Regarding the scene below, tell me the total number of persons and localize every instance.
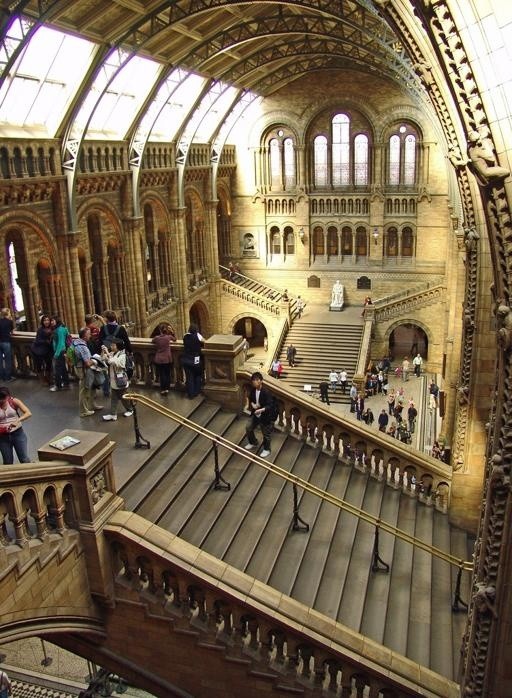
[277,362,283,378]
[319,376,330,405]
[410,332,419,355]
[233,261,244,284]
[0,307,14,381]
[328,367,339,394]
[349,351,441,458]
[363,294,370,305]
[285,343,296,367]
[243,372,274,458]
[242,337,249,362]
[29,308,138,421]
[339,367,348,394]
[330,278,345,306]
[0,386,32,464]
[296,295,304,318]
[263,336,268,351]
[270,358,282,379]
[360,297,373,317]
[228,260,236,283]
[179,321,206,399]
[283,288,289,302]
[150,322,178,395]
[258,361,263,369]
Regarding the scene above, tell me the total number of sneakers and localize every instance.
[92,404,106,411]
[122,410,134,417]
[243,442,259,450]
[48,386,58,392]
[77,409,95,419]
[102,414,118,422]
[259,448,272,458]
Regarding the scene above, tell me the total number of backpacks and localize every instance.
[65,339,87,368]
[102,323,124,353]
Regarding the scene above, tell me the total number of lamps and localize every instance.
[372,226,379,246]
[298,226,305,245]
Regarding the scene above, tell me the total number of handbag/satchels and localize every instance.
[113,371,129,387]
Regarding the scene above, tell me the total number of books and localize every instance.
[49,435,80,451]
